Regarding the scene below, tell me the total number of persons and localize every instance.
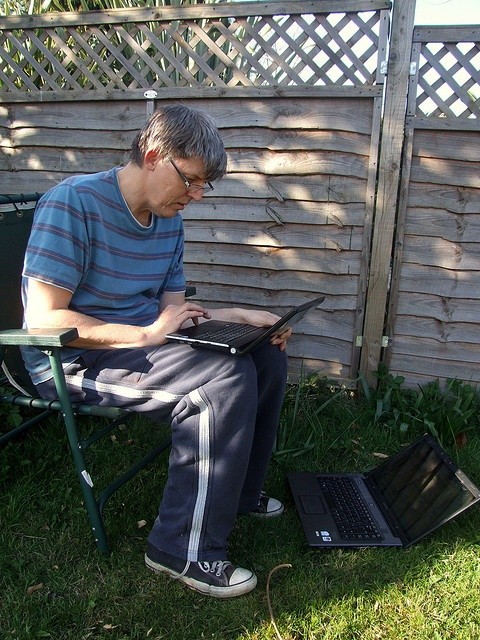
[21,106,293,600]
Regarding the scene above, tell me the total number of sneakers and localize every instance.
[248,495,284,518]
[144,542,258,597]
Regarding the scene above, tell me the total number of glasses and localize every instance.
[169,157,215,197]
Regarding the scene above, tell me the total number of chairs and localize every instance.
[0,193,171,557]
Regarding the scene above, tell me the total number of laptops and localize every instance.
[286,433,479,548]
[161,296,325,353]
